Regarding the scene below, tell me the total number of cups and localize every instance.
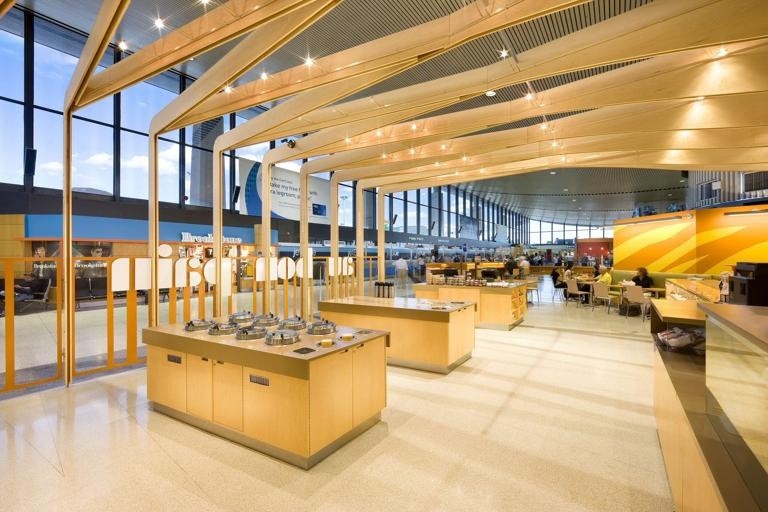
[426,273,487,286]
[744,190,768,199]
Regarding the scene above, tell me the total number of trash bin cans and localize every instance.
[375,282,394,298]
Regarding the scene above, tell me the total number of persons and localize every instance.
[564,264,575,280]
[593,265,600,277]
[520,256,530,267]
[0,270,40,316]
[78,243,107,279]
[582,265,612,305]
[391,256,409,290]
[530,251,613,267]
[631,266,655,286]
[33,244,55,280]
[504,255,519,275]
[416,250,468,276]
[551,267,577,302]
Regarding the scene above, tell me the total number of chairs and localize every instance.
[528,284,539,306]
[375,282,394,297]
[552,277,650,322]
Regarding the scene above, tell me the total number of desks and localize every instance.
[425,262,595,282]
[648,297,707,351]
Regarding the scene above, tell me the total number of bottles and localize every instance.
[374,281,394,298]
